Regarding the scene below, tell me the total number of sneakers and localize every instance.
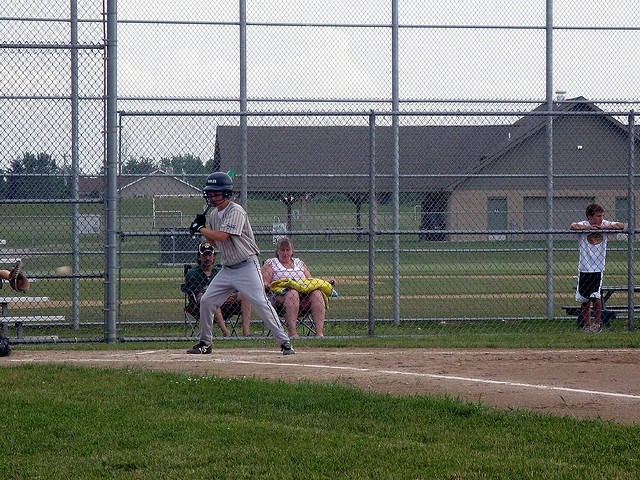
[186,341,212,354]
[582,326,590,331]
[0,341,11,357]
[588,323,602,333]
[281,341,295,354]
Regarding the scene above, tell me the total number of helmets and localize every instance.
[202,172,234,207]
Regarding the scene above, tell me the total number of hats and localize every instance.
[199,242,215,254]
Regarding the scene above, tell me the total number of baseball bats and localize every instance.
[190,169,235,237]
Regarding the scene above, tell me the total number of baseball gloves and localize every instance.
[9,262,29,292]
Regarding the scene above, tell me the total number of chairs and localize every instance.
[262,260,316,336]
[182,262,243,337]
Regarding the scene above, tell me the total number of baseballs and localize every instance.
[58,266,69,277]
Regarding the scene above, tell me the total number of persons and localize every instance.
[260,237,326,338]
[187,243,253,338]
[0,269,22,355]
[570,203,625,334]
[187,172,296,355]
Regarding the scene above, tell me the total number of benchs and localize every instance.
[561,305,640,328]
[571,285,639,308]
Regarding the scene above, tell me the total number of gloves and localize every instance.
[194,214,206,224]
[190,221,204,232]
